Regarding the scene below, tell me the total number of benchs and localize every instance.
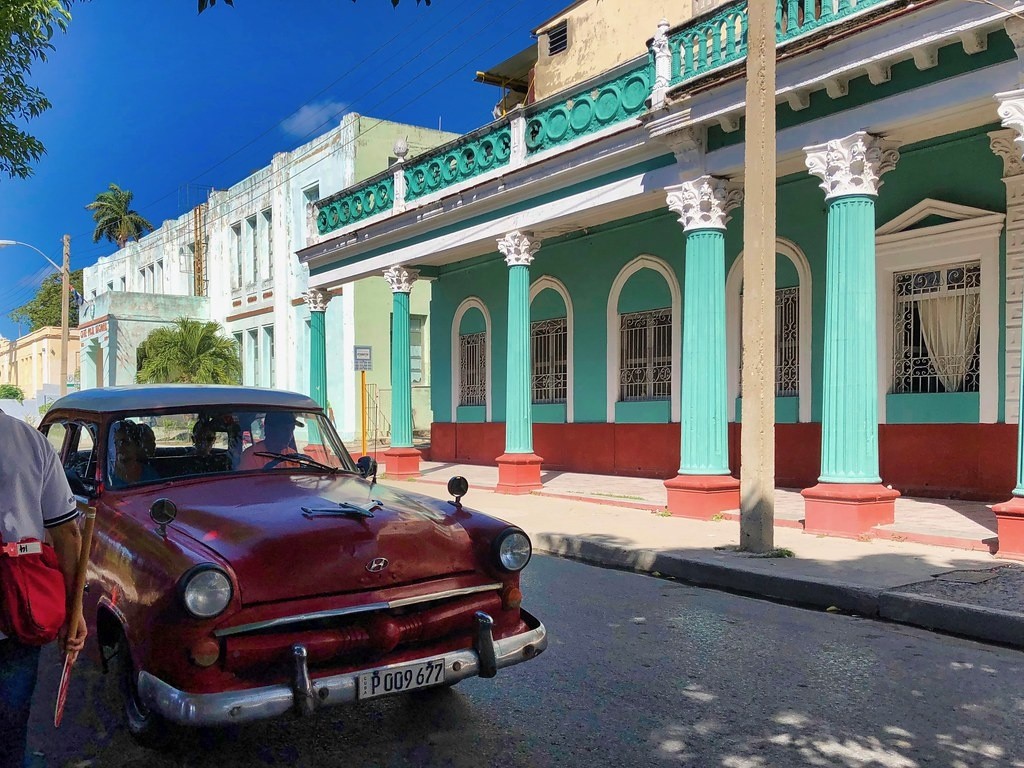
[75,453,232,487]
[69,447,196,469]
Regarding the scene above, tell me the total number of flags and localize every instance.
[73,289,83,305]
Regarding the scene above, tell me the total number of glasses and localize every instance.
[114,438,130,446]
[195,437,214,442]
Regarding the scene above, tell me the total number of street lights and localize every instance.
[0,234,70,398]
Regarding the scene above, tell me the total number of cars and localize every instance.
[37,384,548,748]
[0,399,27,424]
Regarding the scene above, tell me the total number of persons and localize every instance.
[0,409,87,768]
[239,411,305,470]
[173,421,233,477]
[108,420,160,487]
[136,423,156,459]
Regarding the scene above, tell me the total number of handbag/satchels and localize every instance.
[0,537,67,646]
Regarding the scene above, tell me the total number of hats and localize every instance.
[264,411,304,427]
